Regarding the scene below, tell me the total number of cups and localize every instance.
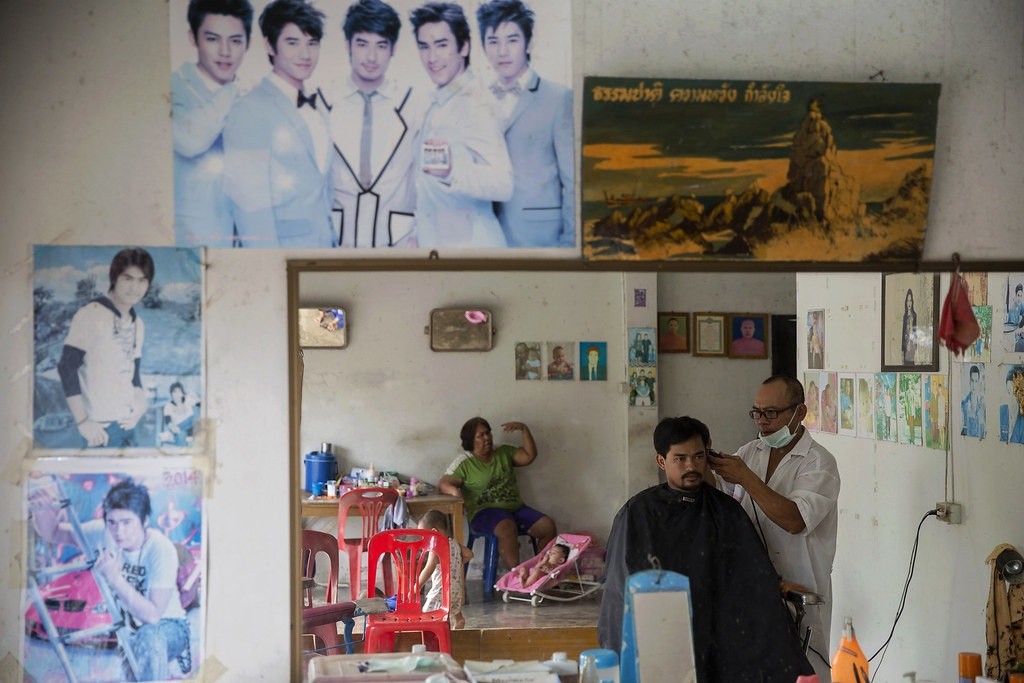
[311,482,325,496]
[321,442,332,454]
[328,480,337,499]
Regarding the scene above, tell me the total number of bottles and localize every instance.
[409,645,426,657]
[828,616,868,683]
[358,465,376,489]
[578,655,601,683]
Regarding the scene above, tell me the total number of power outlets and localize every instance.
[936,502,961,524]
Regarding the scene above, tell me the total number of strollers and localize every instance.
[493,532,602,606]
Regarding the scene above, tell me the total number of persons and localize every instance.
[55,247,196,449]
[28,481,192,682]
[514,343,607,381]
[519,543,570,589]
[407,509,474,629]
[172,0,577,250]
[627,333,657,408]
[440,417,558,570]
[731,318,766,357]
[705,374,841,683]
[597,416,816,683]
[659,318,687,350]
[801,285,1024,448]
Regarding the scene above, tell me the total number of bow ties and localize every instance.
[490,81,522,98]
[297,89,317,109]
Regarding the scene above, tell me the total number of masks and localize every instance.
[759,406,800,449]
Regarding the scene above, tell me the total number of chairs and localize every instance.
[464,519,538,593]
[299,487,453,654]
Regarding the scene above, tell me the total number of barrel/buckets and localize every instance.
[303,451,339,493]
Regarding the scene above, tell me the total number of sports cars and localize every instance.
[24,544,201,650]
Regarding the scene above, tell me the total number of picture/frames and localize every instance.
[658,312,769,360]
[881,272,940,372]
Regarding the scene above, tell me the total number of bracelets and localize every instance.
[76,417,89,425]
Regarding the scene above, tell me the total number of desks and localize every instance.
[301,488,465,606]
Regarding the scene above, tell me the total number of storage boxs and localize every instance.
[308,652,462,683]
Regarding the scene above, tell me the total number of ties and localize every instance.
[357,90,376,190]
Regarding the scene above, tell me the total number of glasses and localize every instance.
[748,403,797,420]
